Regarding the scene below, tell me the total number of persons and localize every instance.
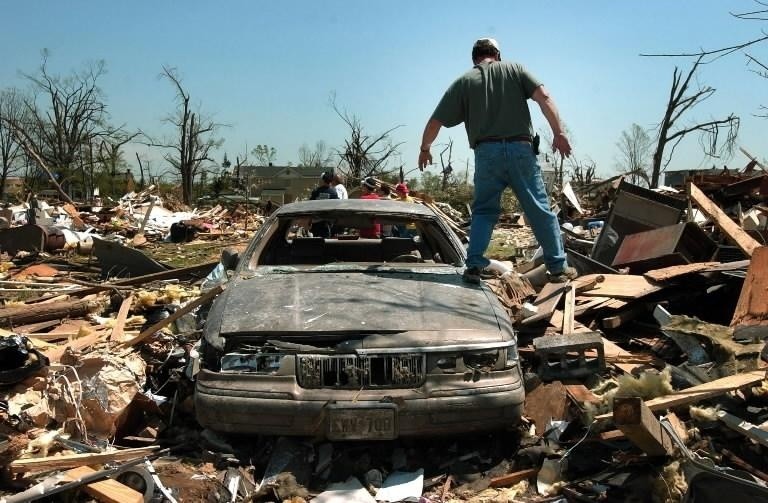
[309,171,348,238]
[418,38,577,285]
[359,177,417,239]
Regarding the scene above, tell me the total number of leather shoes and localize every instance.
[477,135,533,144]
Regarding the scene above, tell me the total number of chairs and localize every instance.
[290,237,337,264]
[379,237,425,264]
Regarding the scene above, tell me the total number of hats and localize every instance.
[473,38,499,51]
[396,184,409,193]
[361,177,376,189]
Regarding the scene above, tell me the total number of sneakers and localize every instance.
[551,267,577,283]
[464,267,480,284]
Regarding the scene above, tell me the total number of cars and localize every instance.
[194,196,527,447]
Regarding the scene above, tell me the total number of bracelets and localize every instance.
[420,146,429,153]
